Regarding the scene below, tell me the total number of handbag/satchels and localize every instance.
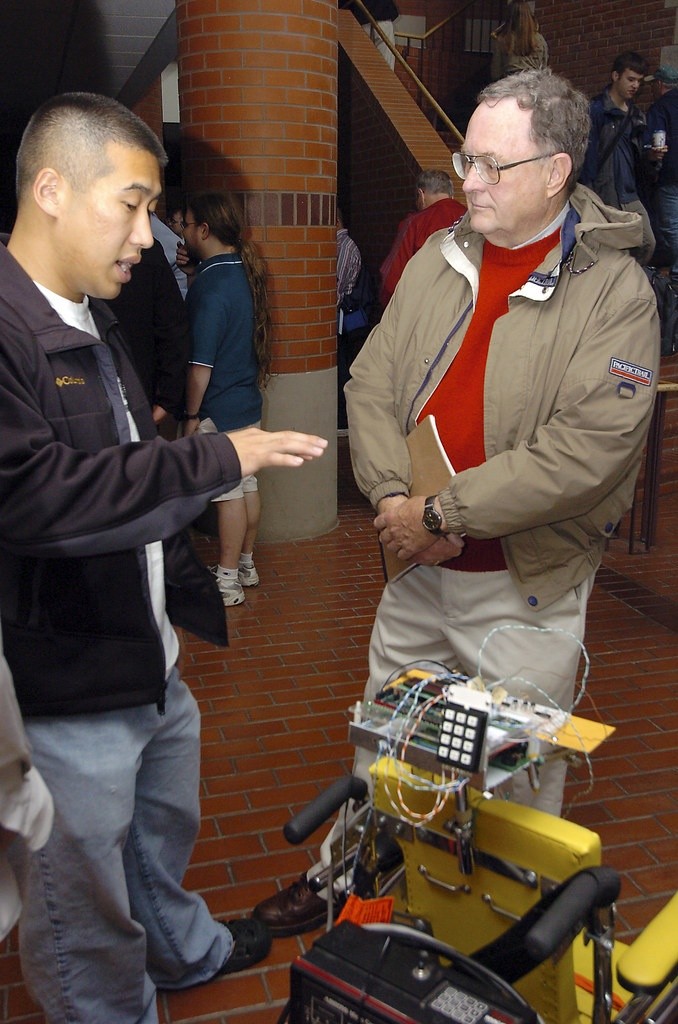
[340,263,376,315]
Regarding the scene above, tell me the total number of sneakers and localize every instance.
[239,561,259,586]
[207,566,245,606]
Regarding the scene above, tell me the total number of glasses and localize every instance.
[452,151,574,185]
[180,222,196,230]
[170,219,178,224]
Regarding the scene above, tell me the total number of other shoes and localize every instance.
[337,429,348,437]
[192,918,273,987]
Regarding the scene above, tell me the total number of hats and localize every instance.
[644,63,678,83]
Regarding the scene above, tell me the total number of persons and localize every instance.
[252,70,661,939]
[1,93,326,1024]
[1,653,55,942]
[105,1,677,606]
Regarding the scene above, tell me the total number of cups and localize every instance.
[653,129,666,147]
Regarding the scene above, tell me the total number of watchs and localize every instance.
[422,494,450,536]
[182,410,199,419]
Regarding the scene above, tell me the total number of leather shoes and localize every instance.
[252,872,342,938]
